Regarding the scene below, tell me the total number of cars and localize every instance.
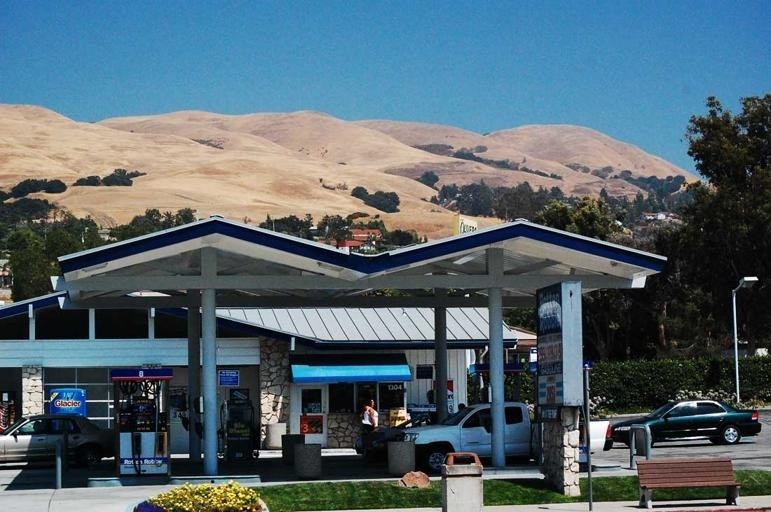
[352,412,437,457]
[0,412,115,469]
[608,399,763,448]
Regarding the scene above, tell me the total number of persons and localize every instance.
[359,399,379,433]
[458,404,465,412]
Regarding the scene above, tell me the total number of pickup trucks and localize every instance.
[404,400,535,474]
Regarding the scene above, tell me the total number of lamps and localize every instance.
[28,304,34,319]
[290,336,296,351]
[150,307,155,317]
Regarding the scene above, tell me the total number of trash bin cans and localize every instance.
[636,424,652,456]
[441,452,484,512]
[281,434,305,465]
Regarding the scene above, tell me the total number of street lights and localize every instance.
[731,275,760,402]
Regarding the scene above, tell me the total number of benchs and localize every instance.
[635,458,742,509]
